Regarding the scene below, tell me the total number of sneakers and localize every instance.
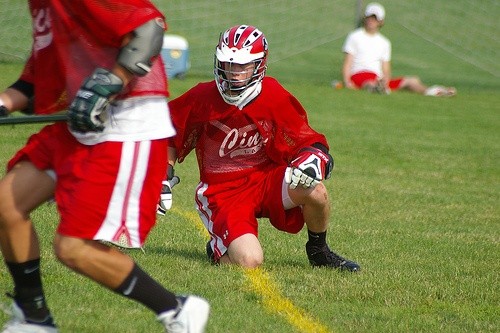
[305,242,360,271]
[0,295,59,333]
[156,296,210,333]
[206,241,220,265]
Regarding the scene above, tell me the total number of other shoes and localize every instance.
[375,79,392,95]
[425,84,455,95]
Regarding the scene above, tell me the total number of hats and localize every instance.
[365,3,387,21]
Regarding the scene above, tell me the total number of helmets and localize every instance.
[214,24,268,91]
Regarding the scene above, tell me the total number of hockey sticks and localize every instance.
[0,110,110,125]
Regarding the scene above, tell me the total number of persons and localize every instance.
[342,1,456,97]
[0,0,214,333]
[156,24,360,272]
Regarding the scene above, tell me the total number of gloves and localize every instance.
[285,146,330,190]
[156,177,181,215]
[67,66,123,133]
